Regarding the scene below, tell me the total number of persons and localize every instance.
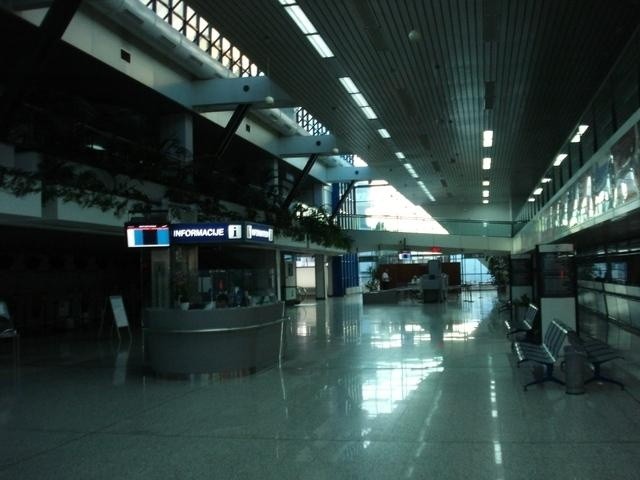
[411,274,420,284]
[203,294,230,309]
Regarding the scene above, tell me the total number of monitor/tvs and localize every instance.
[398,252,411,261]
[124,221,173,250]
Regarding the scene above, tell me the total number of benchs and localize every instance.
[505,303,628,397]
